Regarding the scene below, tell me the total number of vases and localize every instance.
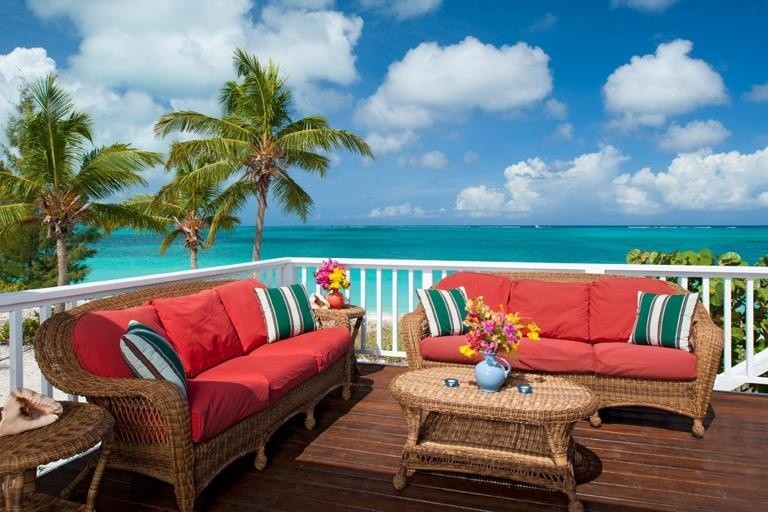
[326,288,344,308]
[473,350,511,393]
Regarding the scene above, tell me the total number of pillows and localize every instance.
[253,283,321,344]
[120,320,187,397]
[628,290,699,353]
[416,285,476,337]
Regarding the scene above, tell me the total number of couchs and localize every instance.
[33,280,351,511]
[398,270,725,437]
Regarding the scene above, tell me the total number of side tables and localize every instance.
[313,304,365,376]
[0,401,115,512]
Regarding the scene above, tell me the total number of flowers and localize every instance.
[312,258,350,292]
[459,297,541,358]
